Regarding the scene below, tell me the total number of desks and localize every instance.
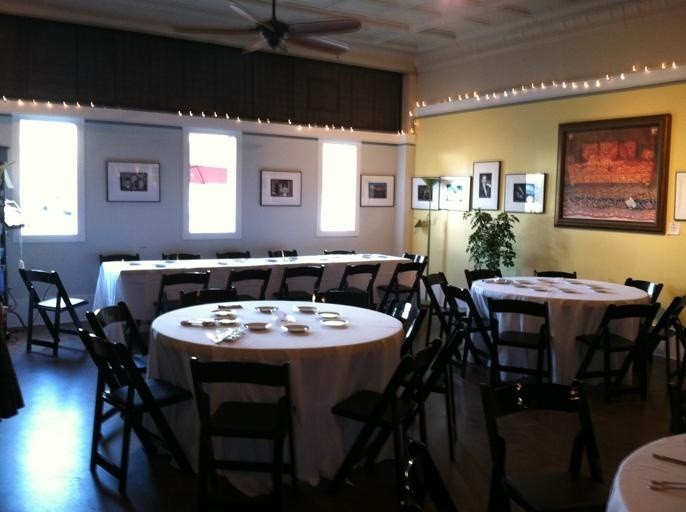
[92,253,416,347]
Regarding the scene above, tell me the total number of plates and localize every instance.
[315,311,342,319]
[539,278,560,286]
[481,277,512,284]
[566,277,587,286]
[587,285,616,295]
[513,282,534,288]
[559,287,584,293]
[243,322,273,330]
[297,306,320,312]
[254,306,278,313]
[515,278,538,285]
[319,319,349,327]
[282,325,310,332]
[532,286,555,293]
[517,279,536,284]
[516,283,528,288]
[210,308,240,316]
[210,316,242,324]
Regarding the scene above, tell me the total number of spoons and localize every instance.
[648,479,686,491]
[588,283,608,294]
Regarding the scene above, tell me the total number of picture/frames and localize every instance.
[259,170,303,207]
[106,159,161,203]
[360,174,395,207]
[554,113,672,235]
[504,172,546,214]
[439,176,473,213]
[411,177,440,211]
[471,161,501,211]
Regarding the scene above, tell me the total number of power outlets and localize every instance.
[666,222,680,235]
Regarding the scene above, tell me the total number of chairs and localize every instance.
[162,251,200,260]
[86,301,147,387]
[376,262,428,322]
[623,278,664,303]
[487,296,553,382]
[399,439,460,512]
[401,327,470,465]
[152,270,211,318]
[220,267,272,302]
[639,294,686,386]
[98,254,141,265]
[401,252,428,309]
[189,356,298,512]
[272,264,325,302]
[18,267,89,357]
[325,249,357,255]
[317,338,443,512]
[439,281,499,382]
[465,268,502,289]
[385,297,428,360]
[215,251,252,259]
[667,315,686,434]
[574,302,661,403]
[267,250,299,257]
[325,262,381,311]
[479,378,611,512]
[78,327,196,495]
[534,270,577,279]
[421,272,466,367]
[180,285,239,307]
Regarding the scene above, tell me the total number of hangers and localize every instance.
[420,177,440,307]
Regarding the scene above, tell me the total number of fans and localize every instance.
[174,1,362,55]
